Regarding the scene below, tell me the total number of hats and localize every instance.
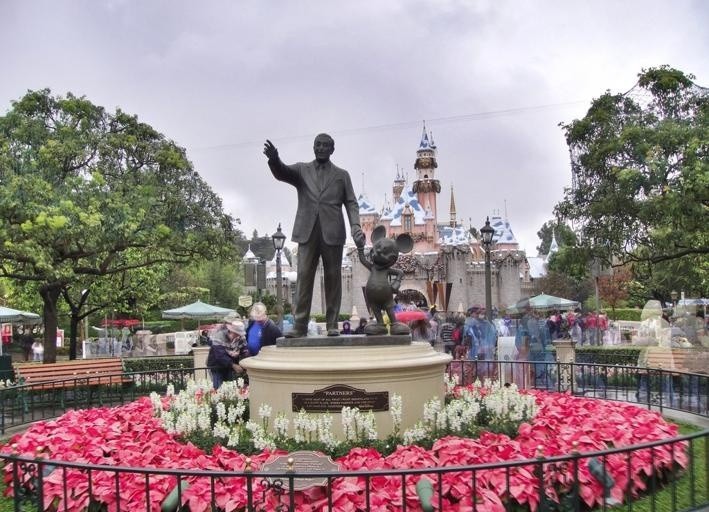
[224,312,246,336]
[250,302,266,317]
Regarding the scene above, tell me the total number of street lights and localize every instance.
[670,290,679,328]
[271,221,287,333]
[479,216,497,322]
[81,287,90,342]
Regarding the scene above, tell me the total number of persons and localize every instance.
[207,319,250,391]
[244,301,283,357]
[355,317,368,334]
[30,337,45,364]
[383,297,708,415]
[262,132,366,340]
[22,330,31,361]
[338,320,355,334]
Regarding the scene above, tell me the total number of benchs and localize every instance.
[13,357,133,414]
[634,348,709,397]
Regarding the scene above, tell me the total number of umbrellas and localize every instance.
[160,301,239,341]
[506,290,582,318]
[0,306,41,357]
